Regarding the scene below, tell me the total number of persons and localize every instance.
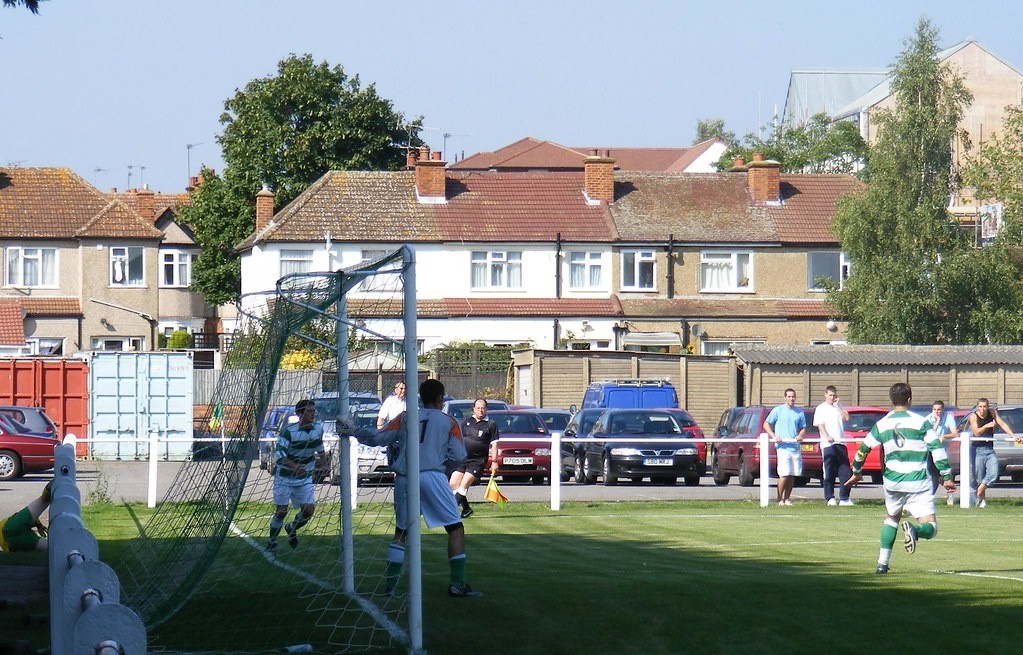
[925,400,957,495]
[968,398,1021,509]
[377,381,420,472]
[449,398,499,518]
[813,386,854,506]
[844,383,957,573]
[336,380,481,597]
[763,389,806,507]
[263,400,331,553]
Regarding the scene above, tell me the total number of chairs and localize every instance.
[613,421,627,432]
[643,422,671,434]
[862,418,873,428]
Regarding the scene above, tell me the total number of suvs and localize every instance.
[943,402,1022,482]
[712,404,823,487]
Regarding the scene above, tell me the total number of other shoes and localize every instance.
[776,498,792,505]
[827,498,837,507]
[975,496,986,508]
[839,497,854,506]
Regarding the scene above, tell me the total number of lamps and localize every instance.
[101,318,108,328]
[357,318,365,327]
[582,320,590,328]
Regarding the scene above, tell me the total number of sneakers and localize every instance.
[901,521,918,554]
[461,507,473,518]
[448,583,483,597]
[384,588,402,597]
[285,523,298,548]
[264,543,279,552]
[875,562,890,574]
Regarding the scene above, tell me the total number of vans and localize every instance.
[571,380,679,438]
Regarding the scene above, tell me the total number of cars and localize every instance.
[0,405,60,478]
[442,396,571,436]
[472,411,552,486]
[582,410,700,487]
[329,409,393,484]
[652,407,707,474]
[712,407,747,454]
[259,392,384,473]
[841,406,892,484]
[561,408,607,481]
[909,404,971,426]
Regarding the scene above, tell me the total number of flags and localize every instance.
[209,401,224,431]
[485,479,508,512]
